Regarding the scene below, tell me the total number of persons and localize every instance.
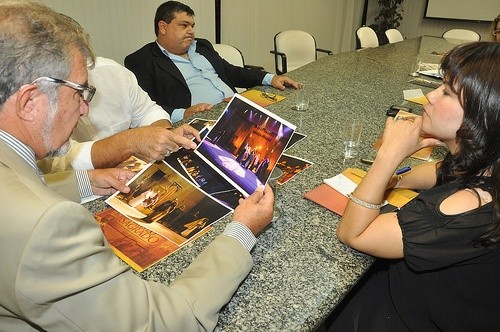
[124,0,301,123]
[36,57,202,173]
[323,41,500,332]
[0,0,273,332]
[491,15,500,42]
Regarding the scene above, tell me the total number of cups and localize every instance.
[409,55,423,76]
[339,123,363,159]
[293,81,310,111]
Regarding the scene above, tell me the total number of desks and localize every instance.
[83,36,473,332]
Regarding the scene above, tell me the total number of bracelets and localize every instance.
[348,193,382,209]
[394,174,402,188]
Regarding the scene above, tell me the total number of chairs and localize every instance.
[212,43,266,96]
[269,28,333,78]
[355,25,480,50]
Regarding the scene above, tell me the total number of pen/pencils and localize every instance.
[397,166,411,175]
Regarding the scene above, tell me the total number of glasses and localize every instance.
[31,76,96,102]
[493,30,500,35]
